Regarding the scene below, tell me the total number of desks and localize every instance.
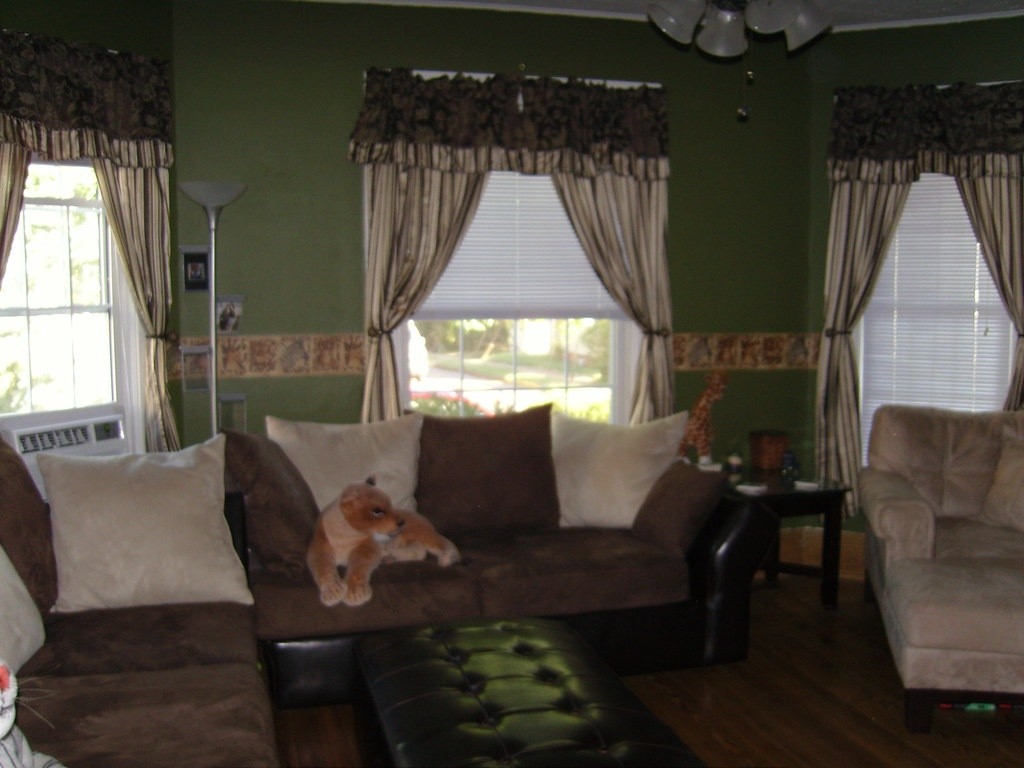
[739,475,854,613]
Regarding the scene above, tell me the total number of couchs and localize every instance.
[17,498,783,768]
[857,408,1024,734]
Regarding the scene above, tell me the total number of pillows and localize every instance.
[218,427,321,588]
[547,410,688,531]
[33,431,255,614]
[629,459,731,559]
[262,410,425,513]
[402,404,561,538]
[0,545,47,677]
[0,433,58,612]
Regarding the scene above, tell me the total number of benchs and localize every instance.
[350,616,707,768]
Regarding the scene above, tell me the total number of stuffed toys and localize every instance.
[308,475,462,607]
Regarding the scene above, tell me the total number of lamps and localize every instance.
[179,181,249,438]
[647,0,835,61]
